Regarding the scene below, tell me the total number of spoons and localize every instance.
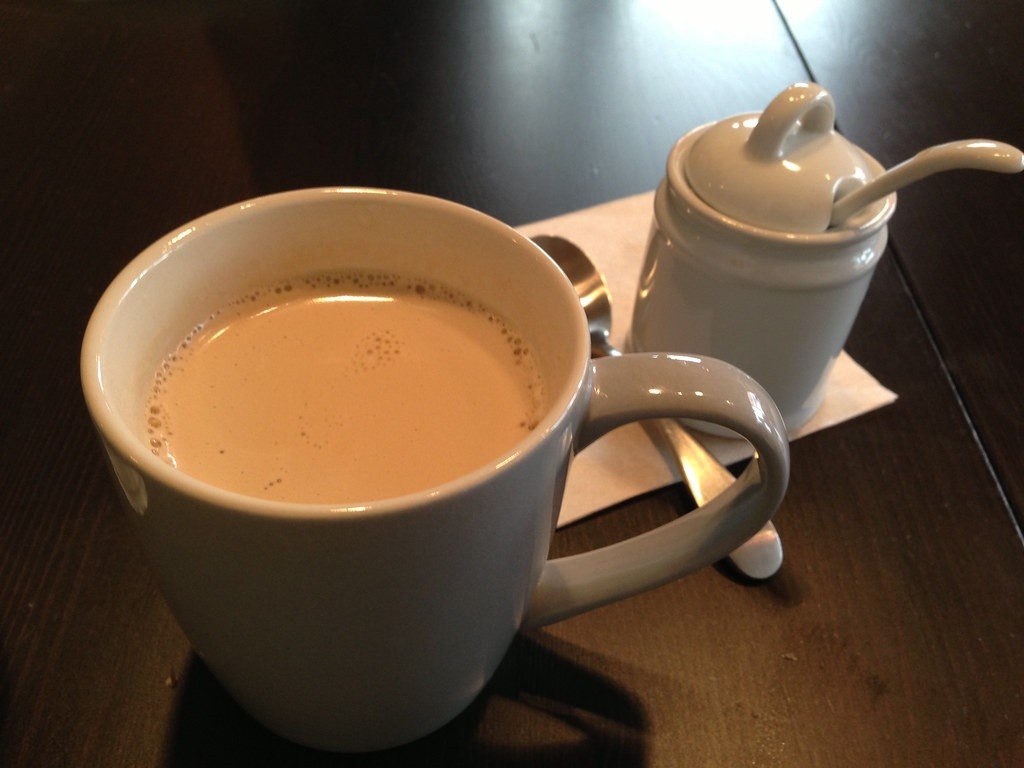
[527,235,783,580]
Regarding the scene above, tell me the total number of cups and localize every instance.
[80,187,791,748]
[632,80,897,438]
